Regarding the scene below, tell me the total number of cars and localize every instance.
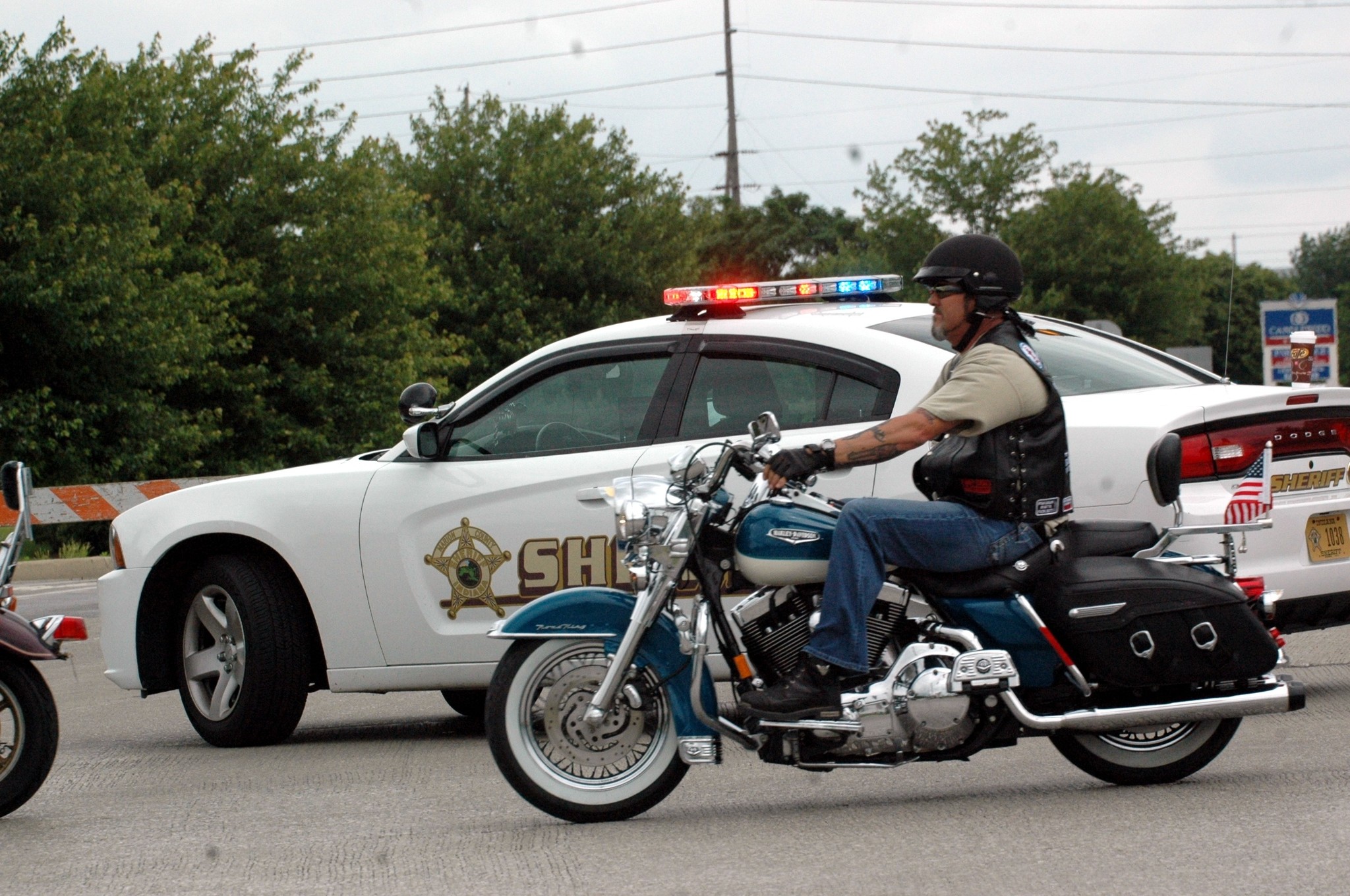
[92,268,1349,740]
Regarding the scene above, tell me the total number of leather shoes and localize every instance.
[740,657,844,722]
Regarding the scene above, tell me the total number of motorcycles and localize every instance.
[466,400,1305,828]
[0,459,84,823]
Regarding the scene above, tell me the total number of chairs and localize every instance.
[696,366,758,434]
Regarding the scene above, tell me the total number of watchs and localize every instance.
[820,438,837,472]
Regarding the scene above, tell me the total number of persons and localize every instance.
[737,234,1073,720]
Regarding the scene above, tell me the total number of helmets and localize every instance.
[912,234,1024,309]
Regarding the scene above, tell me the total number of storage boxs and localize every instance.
[1042,556,1279,691]
